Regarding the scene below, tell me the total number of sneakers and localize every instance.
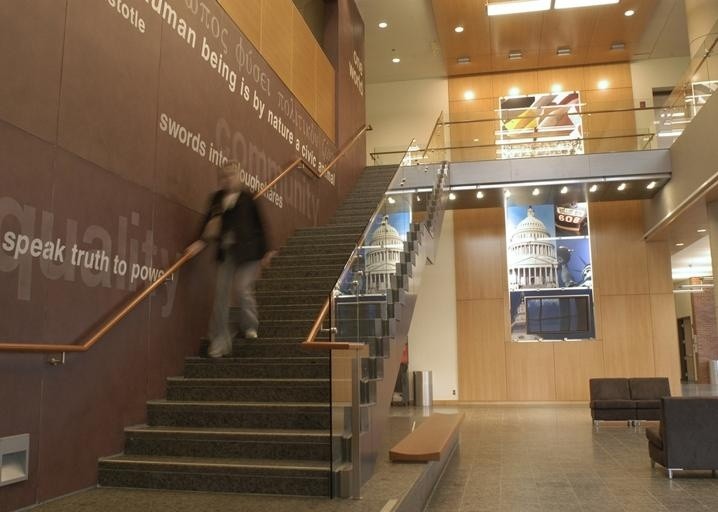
[247,327,258,340]
[209,350,223,358]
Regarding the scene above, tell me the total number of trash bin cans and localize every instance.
[414,371,433,406]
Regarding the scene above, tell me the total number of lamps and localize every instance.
[484,0,621,17]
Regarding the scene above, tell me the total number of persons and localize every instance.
[185,162,276,358]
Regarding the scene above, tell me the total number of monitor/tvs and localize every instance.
[524,294,592,334]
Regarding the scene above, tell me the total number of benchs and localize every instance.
[389,411,464,462]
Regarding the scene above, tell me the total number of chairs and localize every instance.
[590,378,635,426]
[646,398,718,479]
[629,377,670,424]
